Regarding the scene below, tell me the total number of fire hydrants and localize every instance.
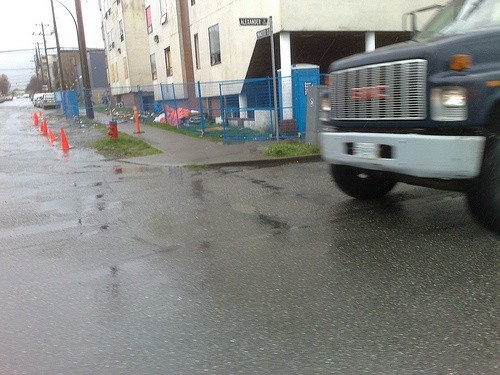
[107,118,120,140]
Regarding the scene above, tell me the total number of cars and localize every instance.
[31,92,57,111]
[0,93,12,104]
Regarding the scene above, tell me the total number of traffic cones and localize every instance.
[33,111,50,136]
[47,127,59,143]
[59,127,76,151]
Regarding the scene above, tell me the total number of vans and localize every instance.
[316,0,500,238]
